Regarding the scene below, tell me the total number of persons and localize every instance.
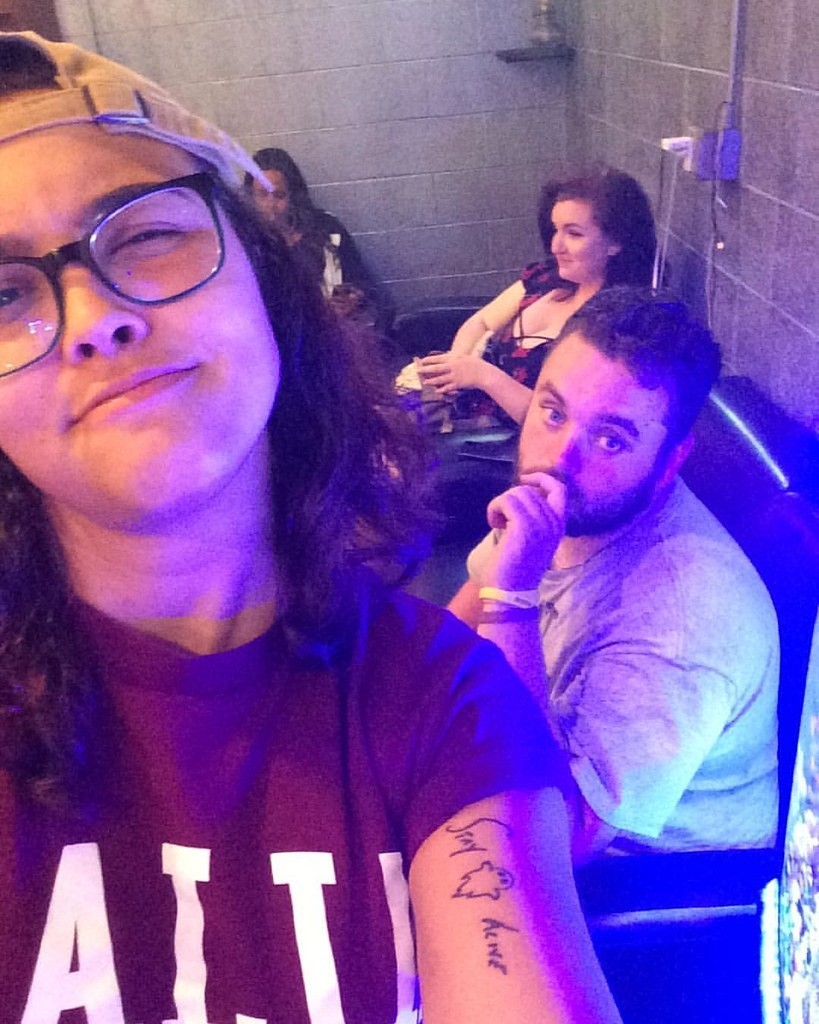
[395,160,657,435]
[241,147,395,350]
[441,287,780,872]
[0,29,620,1023]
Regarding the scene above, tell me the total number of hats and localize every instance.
[0,29,276,197]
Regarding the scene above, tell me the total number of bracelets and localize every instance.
[476,587,541,624]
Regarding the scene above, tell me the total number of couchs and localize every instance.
[383,293,819,1024]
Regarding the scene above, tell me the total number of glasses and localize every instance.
[0,173,223,379]
